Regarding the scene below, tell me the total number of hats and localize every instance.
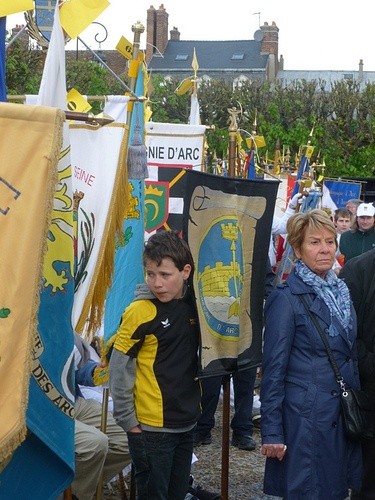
[357,203,375,216]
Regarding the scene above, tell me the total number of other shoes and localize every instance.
[191,476,221,500]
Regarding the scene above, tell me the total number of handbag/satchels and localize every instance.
[340,384,375,441]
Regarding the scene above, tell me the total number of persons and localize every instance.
[63,199,375,500]
[260,209,362,500]
[108,230,202,500]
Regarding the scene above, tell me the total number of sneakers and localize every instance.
[199,428,213,444]
[231,434,256,449]
